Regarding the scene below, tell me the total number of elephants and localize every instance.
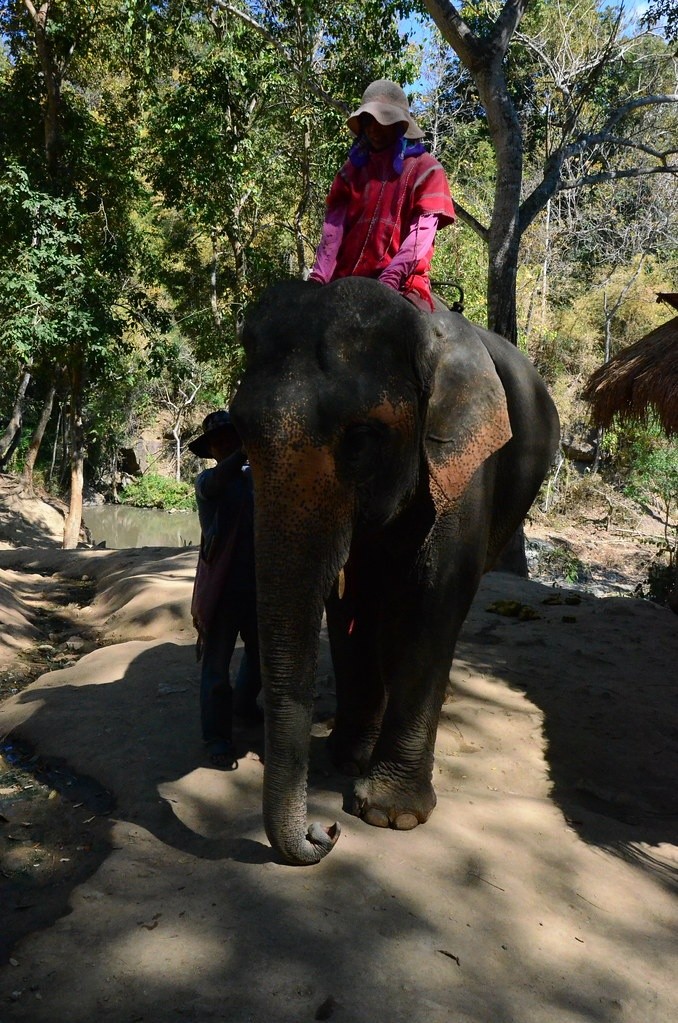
[229,276,559,867]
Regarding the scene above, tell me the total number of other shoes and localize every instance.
[205,741,236,768]
[234,701,265,722]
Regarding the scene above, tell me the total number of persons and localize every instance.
[308,78,454,313]
[188,411,264,766]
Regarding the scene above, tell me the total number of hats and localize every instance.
[188,411,236,459]
[346,80,427,140]
